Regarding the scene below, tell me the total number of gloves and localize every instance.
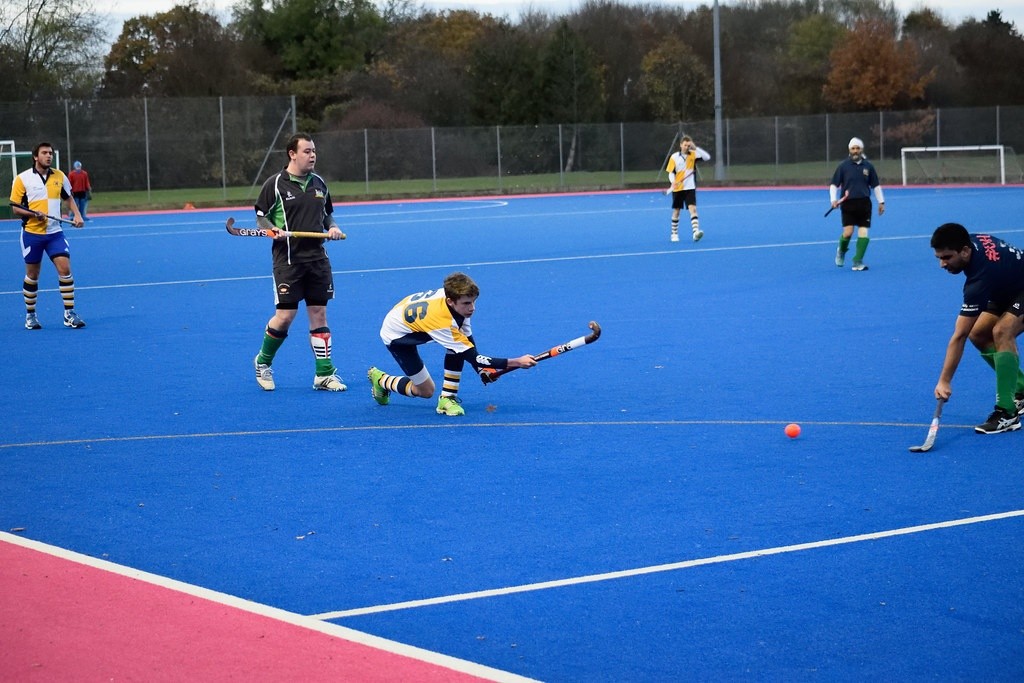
[477,366,500,384]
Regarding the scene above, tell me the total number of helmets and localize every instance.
[74,161,82,168]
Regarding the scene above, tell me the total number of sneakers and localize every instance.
[836,246,846,266]
[313,368,348,392]
[975,405,1022,434]
[851,262,868,270]
[63,309,86,328]
[693,230,704,242]
[436,394,465,416]
[670,233,680,242]
[1012,393,1024,414]
[26,312,43,330]
[254,353,275,391]
[367,367,392,406]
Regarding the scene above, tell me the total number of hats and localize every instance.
[848,136,864,153]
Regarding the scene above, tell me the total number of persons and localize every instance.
[255,133,348,392]
[931,223,1024,434]
[828,137,884,271]
[10,143,85,330]
[666,136,711,242]
[368,271,539,417]
[68,161,92,221]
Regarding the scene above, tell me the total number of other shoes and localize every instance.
[82,217,90,221]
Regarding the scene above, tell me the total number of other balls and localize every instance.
[784,424,801,438]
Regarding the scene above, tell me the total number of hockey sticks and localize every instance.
[225,216,347,240]
[907,396,944,452]
[824,189,849,218]
[662,170,695,196]
[8,202,77,225]
[481,320,601,384]
